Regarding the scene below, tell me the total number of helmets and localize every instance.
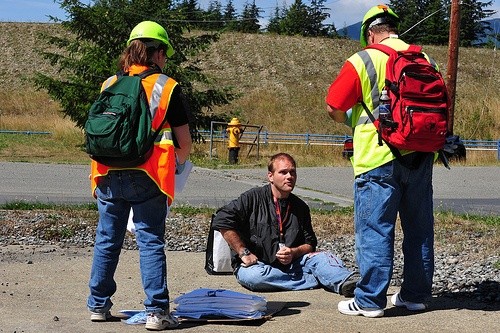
[360,4,399,48]
[127,21,174,59]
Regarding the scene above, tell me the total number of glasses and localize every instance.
[164,53,169,63]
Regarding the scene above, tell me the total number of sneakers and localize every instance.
[342,274,362,297]
[338,298,384,318]
[90,310,112,321]
[145,311,179,331]
[391,292,428,310]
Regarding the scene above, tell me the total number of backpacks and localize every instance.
[361,43,449,151]
[84,69,168,168]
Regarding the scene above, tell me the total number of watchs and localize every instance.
[239,248,250,258]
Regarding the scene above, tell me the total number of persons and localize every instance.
[327,5,440,318]
[225,118,244,163]
[87,20,192,330]
[211,153,359,297]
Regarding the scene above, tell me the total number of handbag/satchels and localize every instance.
[204,214,234,275]
